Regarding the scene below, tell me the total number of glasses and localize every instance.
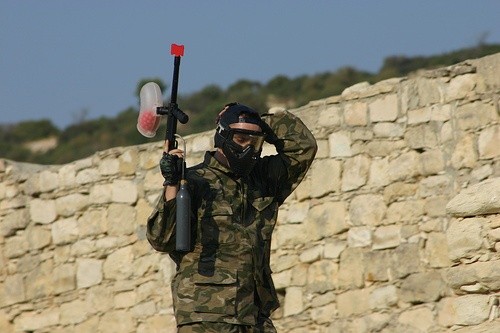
[219,122,264,153]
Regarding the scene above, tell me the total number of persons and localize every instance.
[146,102,319,333]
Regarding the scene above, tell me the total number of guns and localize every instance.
[137,43,192,251]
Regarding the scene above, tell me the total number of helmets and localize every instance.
[213,102,266,147]
